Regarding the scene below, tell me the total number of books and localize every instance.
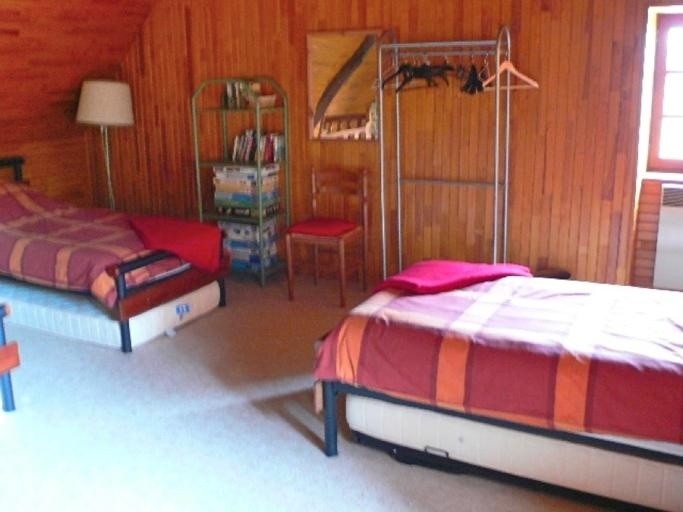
[208,81,282,160]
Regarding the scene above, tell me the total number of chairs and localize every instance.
[284,167,369,310]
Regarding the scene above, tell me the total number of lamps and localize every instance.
[76,80,136,212]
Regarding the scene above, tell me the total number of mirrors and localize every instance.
[305,28,383,142]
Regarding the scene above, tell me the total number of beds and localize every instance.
[315,269,683,512]
[0,156,228,352]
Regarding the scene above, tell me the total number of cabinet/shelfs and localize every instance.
[191,76,293,290]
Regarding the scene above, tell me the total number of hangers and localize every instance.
[371,49,540,95]
